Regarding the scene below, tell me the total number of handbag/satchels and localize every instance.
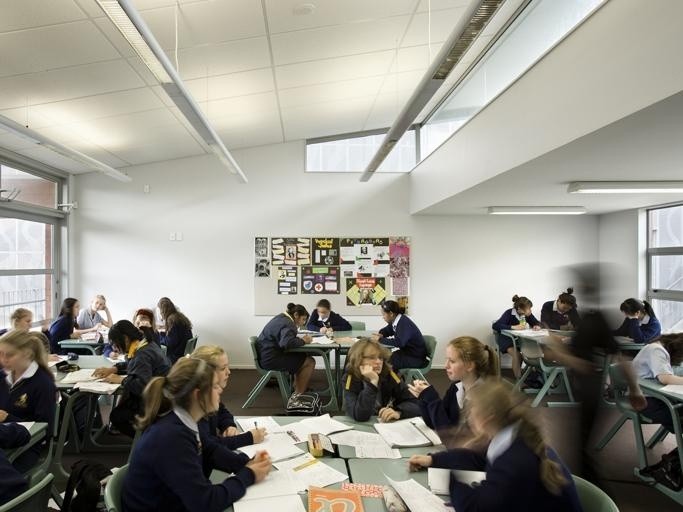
[521,369,545,389]
[640,456,682,492]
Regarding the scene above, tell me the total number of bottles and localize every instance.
[67,352,79,371]
[519,313,526,326]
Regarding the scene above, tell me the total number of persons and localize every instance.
[252,300,316,394]
[627,331,683,494]
[359,246,367,254]
[408,336,502,448]
[46,297,83,355]
[537,286,579,330]
[104,297,197,436]
[490,291,550,388]
[367,299,429,373]
[124,342,274,510]
[0,330,59,480]
[285,246,295,260]
[76,295,116,328]
[403,374,578,511]
[1,307,34,340]
[604,299,663,395]
[541,259,648,505]
[339,337,422,423]
[0,420,42,511]
[304,298,352,333]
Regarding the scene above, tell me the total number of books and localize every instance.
[228,414,490,511]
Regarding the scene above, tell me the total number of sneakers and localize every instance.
[602,388,618,405]
[287,392,311,409]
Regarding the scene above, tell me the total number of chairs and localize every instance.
[0,335,198,510]
[491,320,681,511]
[242,320,436,410]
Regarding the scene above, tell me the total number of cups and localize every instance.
[307,433,324,457]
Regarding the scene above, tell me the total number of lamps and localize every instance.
[566,181,682,193]
[487,206,587,215]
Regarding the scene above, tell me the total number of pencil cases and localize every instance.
[308,433,335,457]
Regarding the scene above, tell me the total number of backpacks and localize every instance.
[61,464,113,512]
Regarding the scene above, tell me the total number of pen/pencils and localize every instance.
[254,422,258,430]
[323,323,326,328]
